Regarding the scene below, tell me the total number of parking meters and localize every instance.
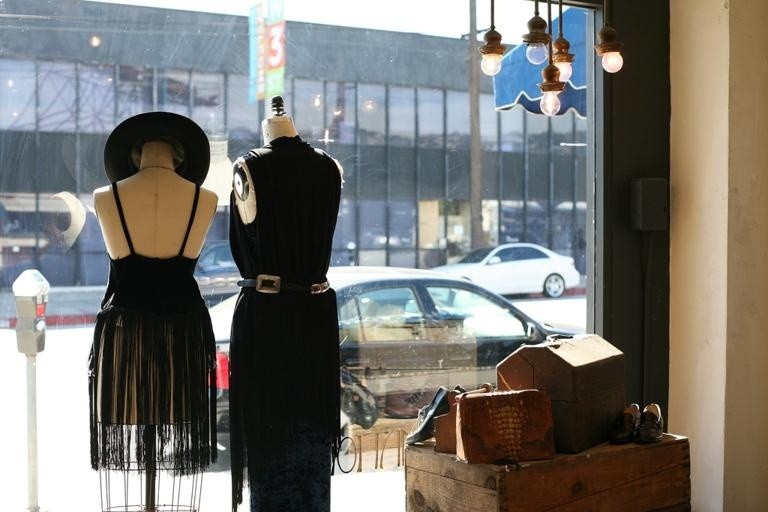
[12,268,53,512]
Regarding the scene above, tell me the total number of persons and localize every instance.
[223,108,347,512]
[85,111,221,476]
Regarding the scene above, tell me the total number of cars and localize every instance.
[207,265,575,450]
[360,223,404,250]
[194,237,244,302]
[427,243,580,298]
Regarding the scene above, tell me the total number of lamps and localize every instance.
[477,1,626,119]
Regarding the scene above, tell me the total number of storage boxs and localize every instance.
[495,332,629,455]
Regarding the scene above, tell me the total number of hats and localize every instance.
[104,111,210,187]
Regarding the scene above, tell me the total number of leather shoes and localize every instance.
[405,386,468,445]
[384,389,436,417]
[352,398,378,429]
[637,402,664,443]
[609,403,640,444]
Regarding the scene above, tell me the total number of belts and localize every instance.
[237,276,329,297]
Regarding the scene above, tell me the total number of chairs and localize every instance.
[338,299,405,345]
